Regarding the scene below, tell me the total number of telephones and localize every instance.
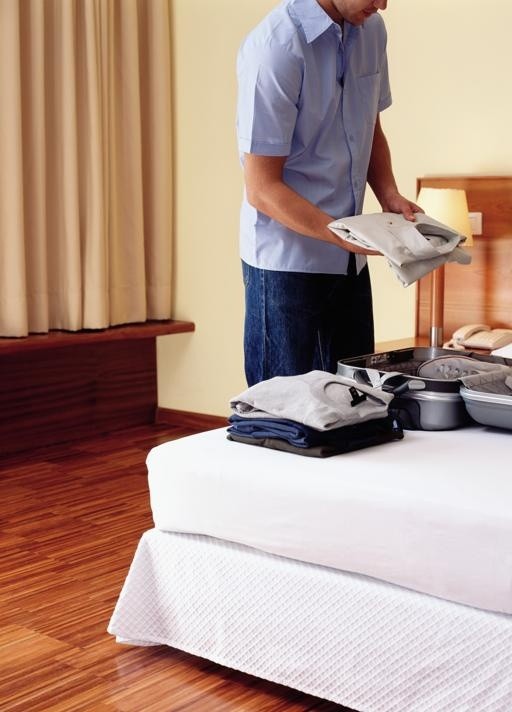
[452,324,512,350]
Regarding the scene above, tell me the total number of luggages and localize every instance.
[336,328,512,431]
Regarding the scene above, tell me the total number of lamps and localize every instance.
[412,188,474,361]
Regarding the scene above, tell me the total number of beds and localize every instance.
[105,176,512,712]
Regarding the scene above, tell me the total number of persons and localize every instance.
[235,0,425,387]
